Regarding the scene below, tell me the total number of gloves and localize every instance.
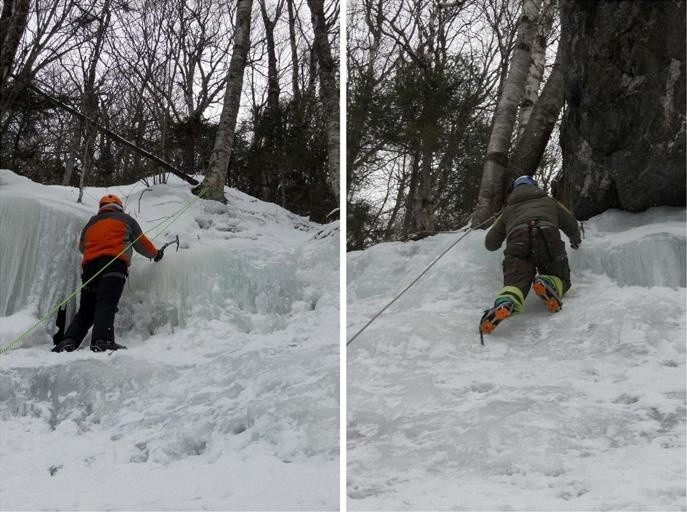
[154,251,165,261]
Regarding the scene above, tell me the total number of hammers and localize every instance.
[161,234,180,252]
[570,222,585,248]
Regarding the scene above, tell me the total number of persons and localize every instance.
[50,194,163,353]
[478,175,581,334]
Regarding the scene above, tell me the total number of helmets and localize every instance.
[513,175,537,186]
[100,195,122,208]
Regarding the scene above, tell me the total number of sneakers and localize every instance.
[91,340,127,352]
[51,339,75,352]
[532,277,562,312]
[480,301,513,333]
